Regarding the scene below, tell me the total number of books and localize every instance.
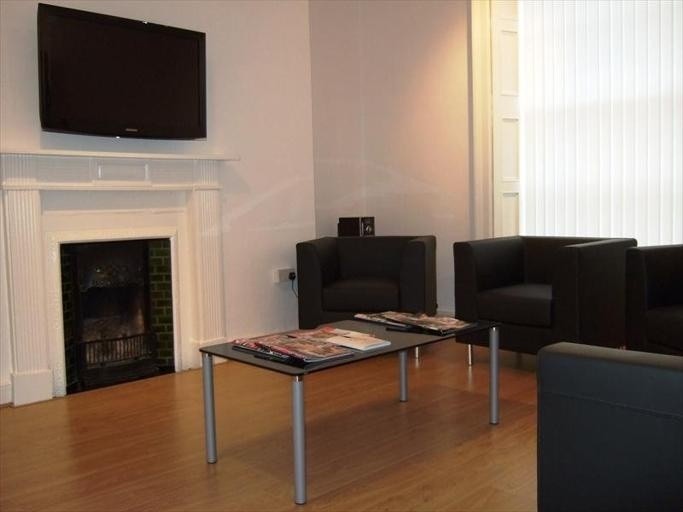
[230,310,477,365]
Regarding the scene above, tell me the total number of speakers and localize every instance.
[338,216,375,237]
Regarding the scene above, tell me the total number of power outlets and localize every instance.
[274,267,296,283]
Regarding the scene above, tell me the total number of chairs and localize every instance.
[296,236,436,328]
[536,341,682,512]
[453,234,637,366]
[626,243,682,355]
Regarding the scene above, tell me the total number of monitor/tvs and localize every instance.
[36,3,206,141]
